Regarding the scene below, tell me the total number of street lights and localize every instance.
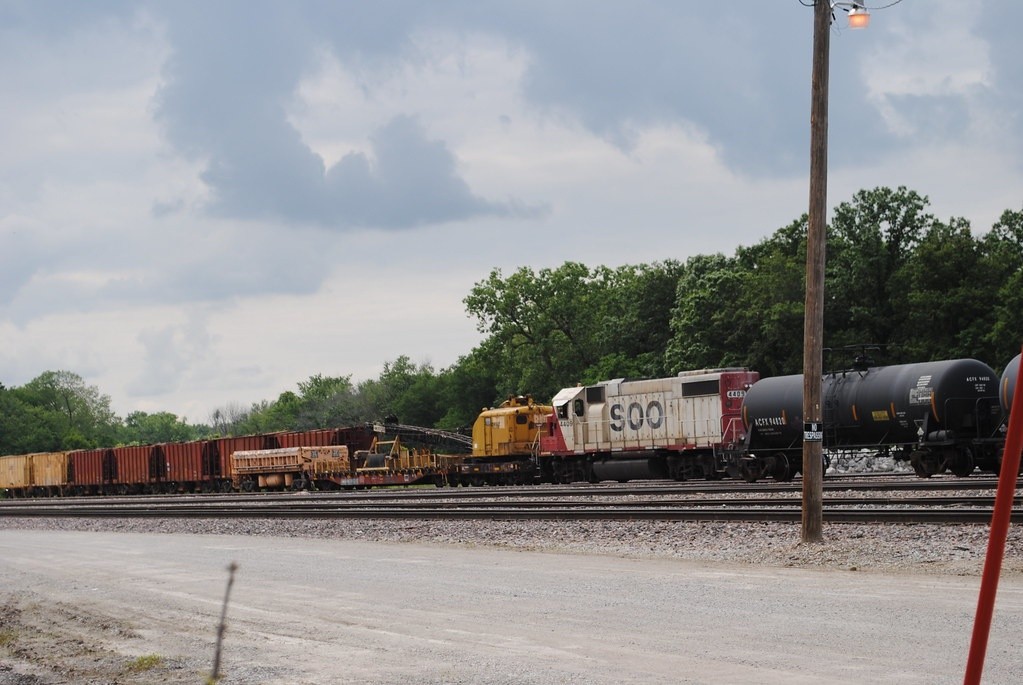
[800,0,873,544]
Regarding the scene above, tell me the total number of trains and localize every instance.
[4,395,554,487]
[536,344,1023,485]
